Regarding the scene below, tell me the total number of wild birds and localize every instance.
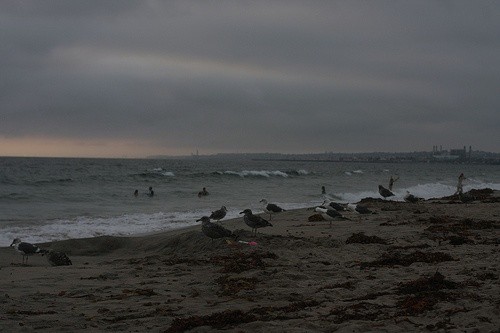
[320,199,357,214]
[209,205,228,221]
[9,236,51,265]
[403,189,424,203]
[238,209,274,236]
[198,186,209,199]
[379,180,395,200]
[135,186,154,196]
[259,198,288,222]
[196,215,232,243]
[314,206,355,227]
